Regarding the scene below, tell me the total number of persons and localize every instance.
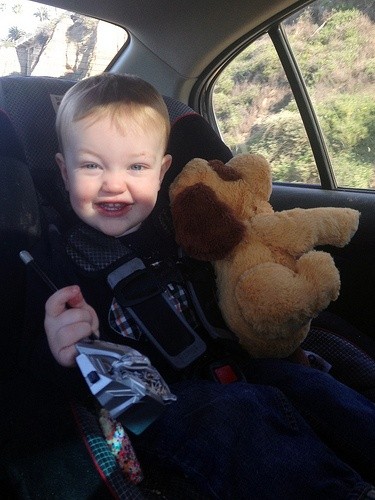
[0,71,374,500]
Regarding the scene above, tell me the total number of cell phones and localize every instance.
[129,293,208,370]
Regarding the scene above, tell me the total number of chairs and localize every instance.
[2,74,374,500]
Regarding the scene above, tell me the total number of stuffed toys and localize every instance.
[167,152,362,360]
